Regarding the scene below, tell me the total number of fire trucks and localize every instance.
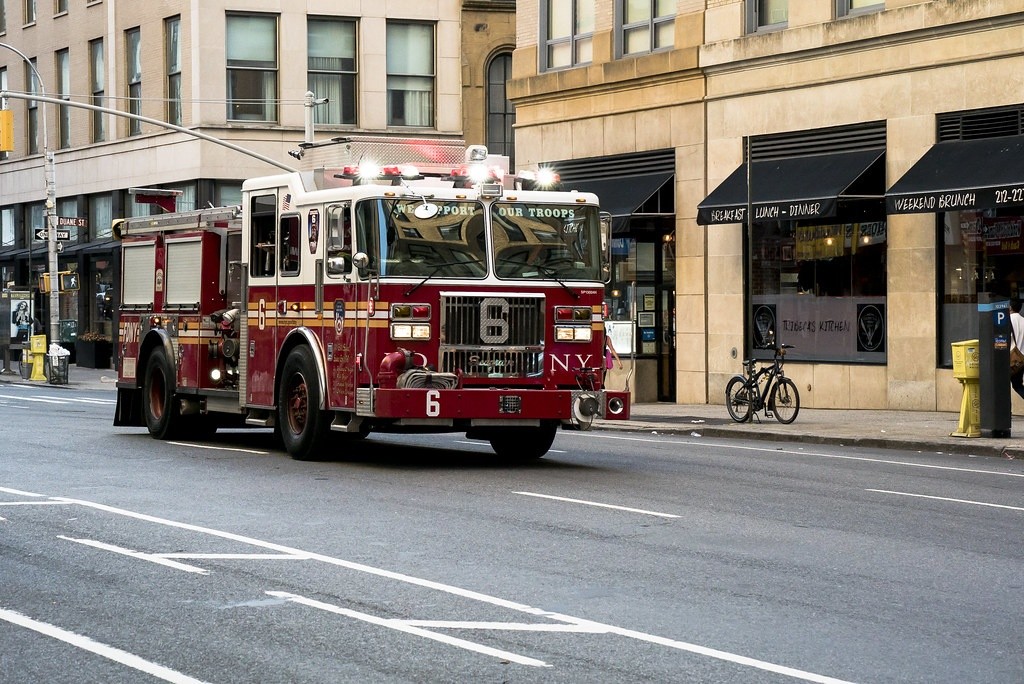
[107,128,636,462]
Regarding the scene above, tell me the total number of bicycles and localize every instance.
[725,331,801,424]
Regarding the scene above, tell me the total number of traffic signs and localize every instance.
[57,215,88,227]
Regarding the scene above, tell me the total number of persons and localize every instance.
[309,224,318,254]
[1007,296,1024,399]
[600,327,624,389]
[12,301,34,344]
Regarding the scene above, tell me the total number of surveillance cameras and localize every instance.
[314,98,329,105]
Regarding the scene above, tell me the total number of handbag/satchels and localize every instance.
[605,349,613,369]
[1010,346,1024,375]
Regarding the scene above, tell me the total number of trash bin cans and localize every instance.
[46,353,69,386]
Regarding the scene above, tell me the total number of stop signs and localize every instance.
[35,228,72,241]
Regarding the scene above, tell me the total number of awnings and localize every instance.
[0,239,122,260]
[696,149,886,225]
[882,134,1024,215]
[514,173,673,234]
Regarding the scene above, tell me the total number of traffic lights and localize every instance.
[60,272,81,292]
[36,276,51,295]
